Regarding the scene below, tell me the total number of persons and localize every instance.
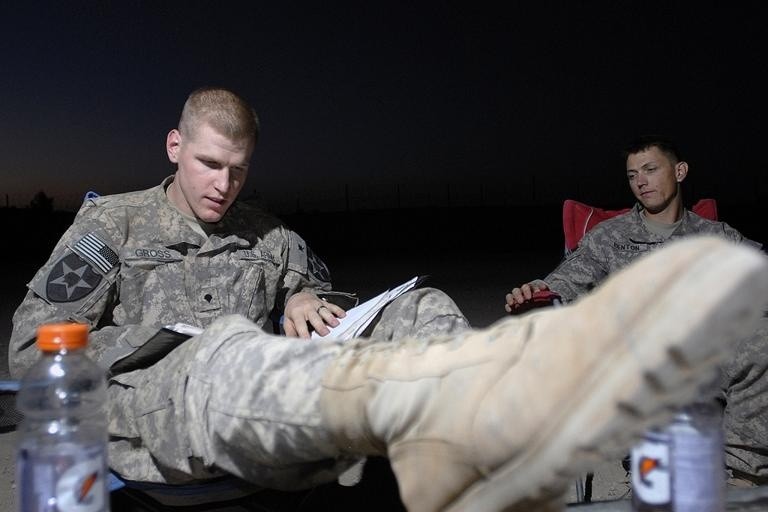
[503,135,768,510]
[7,86,765,511]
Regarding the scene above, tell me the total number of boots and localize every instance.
[323,234,767,511]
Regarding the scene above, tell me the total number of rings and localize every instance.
[316,306,328,314]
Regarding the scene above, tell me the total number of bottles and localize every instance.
[14,321,112,512]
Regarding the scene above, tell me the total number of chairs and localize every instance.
[508,198,716,316]
